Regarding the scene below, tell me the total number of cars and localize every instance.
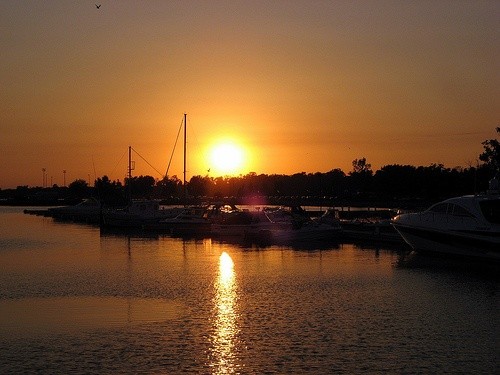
[388,194,499,233]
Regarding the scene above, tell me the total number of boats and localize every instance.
[23,198,342,240]
